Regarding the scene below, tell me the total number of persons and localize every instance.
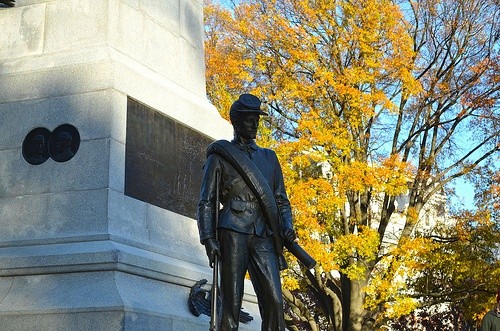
[483,290,500,331]
[194,93,296,331]
